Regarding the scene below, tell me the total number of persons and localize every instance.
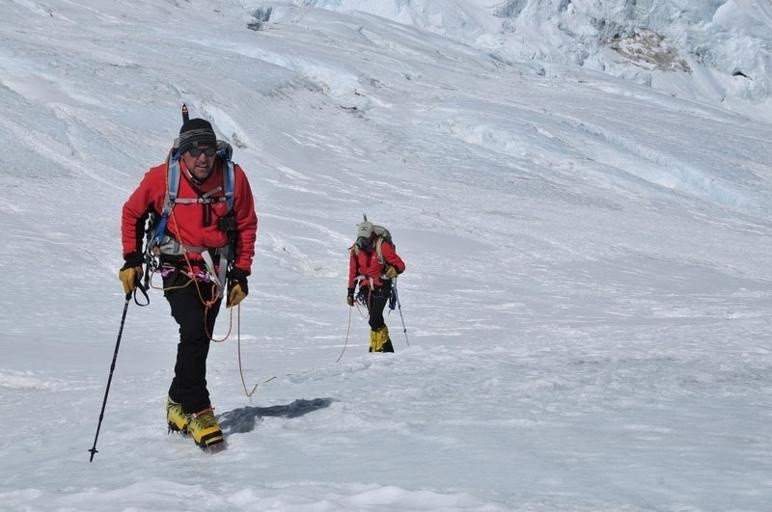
[119,118,258,449]
[346,221,405,354]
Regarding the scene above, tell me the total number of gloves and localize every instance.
[385,265,398,278]
[119,258,146,294]
[226,266,249,309]
[347,293,356,306]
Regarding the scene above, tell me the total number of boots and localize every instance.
[369,326,393,353]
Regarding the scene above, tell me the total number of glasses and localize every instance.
[187,146,217,157]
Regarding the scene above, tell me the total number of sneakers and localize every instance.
[165,393,191,431]
[187,407,224,450]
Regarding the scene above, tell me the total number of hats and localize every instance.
[178,118,217,154]
[358,221,375,238]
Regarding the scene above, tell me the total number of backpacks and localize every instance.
[354,225,395,268]
[155,137,236,220]
